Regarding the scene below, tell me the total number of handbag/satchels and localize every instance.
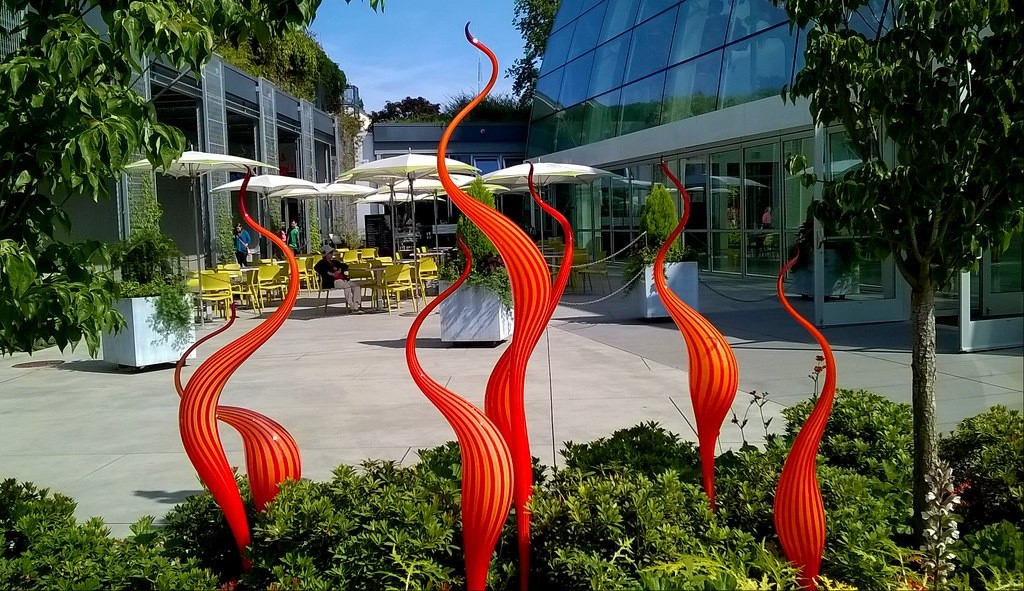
[247,248,250,255]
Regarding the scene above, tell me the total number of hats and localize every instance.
[320,245,334,257]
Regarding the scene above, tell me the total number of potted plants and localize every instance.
[617,181,698,322]
[100,176,198,373]
[439,177,513,346]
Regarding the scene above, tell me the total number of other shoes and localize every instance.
[352,309,364,314]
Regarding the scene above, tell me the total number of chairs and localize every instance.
[186,246,441,321]
[536,237,613,298]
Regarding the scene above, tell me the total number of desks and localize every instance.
[433,247,452,265]
[344,266,415,312]
[395,259,419,264]
[544,255,564,286]
[409,253,445,258]
[240,266,285,309]
[206,275,239,320]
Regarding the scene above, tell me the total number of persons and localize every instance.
[286,219,301,255]
[271,225,287,261]
[233,223,251,267]
[314,244,366,315]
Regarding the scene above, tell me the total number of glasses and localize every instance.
[329,251,333,254]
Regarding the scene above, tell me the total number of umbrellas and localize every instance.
[123,143,281,328]
[324,146,545,259]
[456,156,620,261]
[209,169,319,257]
[566,172,770,251]
[260,178,380,252]
[783,157,879,181]
